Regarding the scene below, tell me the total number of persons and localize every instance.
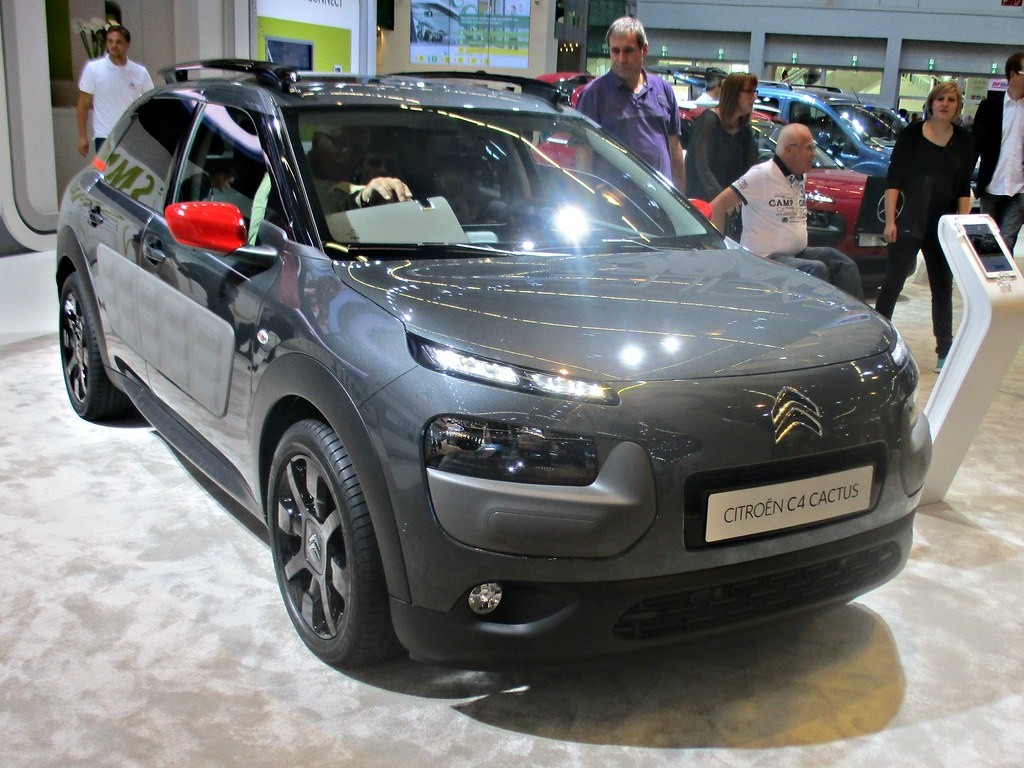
[873,81,971,374]
[960,115,974,131]
[77,24,155,159]
[567,16,686,203]
[178,166,238,202]
[694,67,728,103]
[684,72,760,238]
[429,152,527,242]
[708,122,865,306]
[898,109,920,125]
[298,117,414,214]
[970,52,1024,259]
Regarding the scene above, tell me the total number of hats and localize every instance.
[704,67,729,79]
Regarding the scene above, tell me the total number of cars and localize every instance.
[534,64,941,261]
[54,52,939,674]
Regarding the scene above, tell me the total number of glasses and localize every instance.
[743,89,758,94]
[315,131,364,156]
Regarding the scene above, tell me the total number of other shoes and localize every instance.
[935,354,948,373]
